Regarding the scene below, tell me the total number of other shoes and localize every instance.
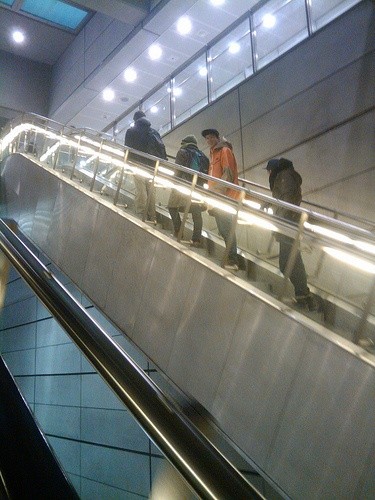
[223,256,239,271]
[190,239,202,249]
[170,232,184,239]
[293,295,319,312]
[145,214,157,224]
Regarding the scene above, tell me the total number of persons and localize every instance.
[201,129,241,269]
[124,110,160,226]
[263,156,319,312]
[167,135,210,247]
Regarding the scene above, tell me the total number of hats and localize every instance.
[133,110,146,120]
[181,134,200,146]
[262,158,279,169]
[201,129,220,138]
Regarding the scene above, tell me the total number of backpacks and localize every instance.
[134,125,168,161]
[185,147,209,175]
